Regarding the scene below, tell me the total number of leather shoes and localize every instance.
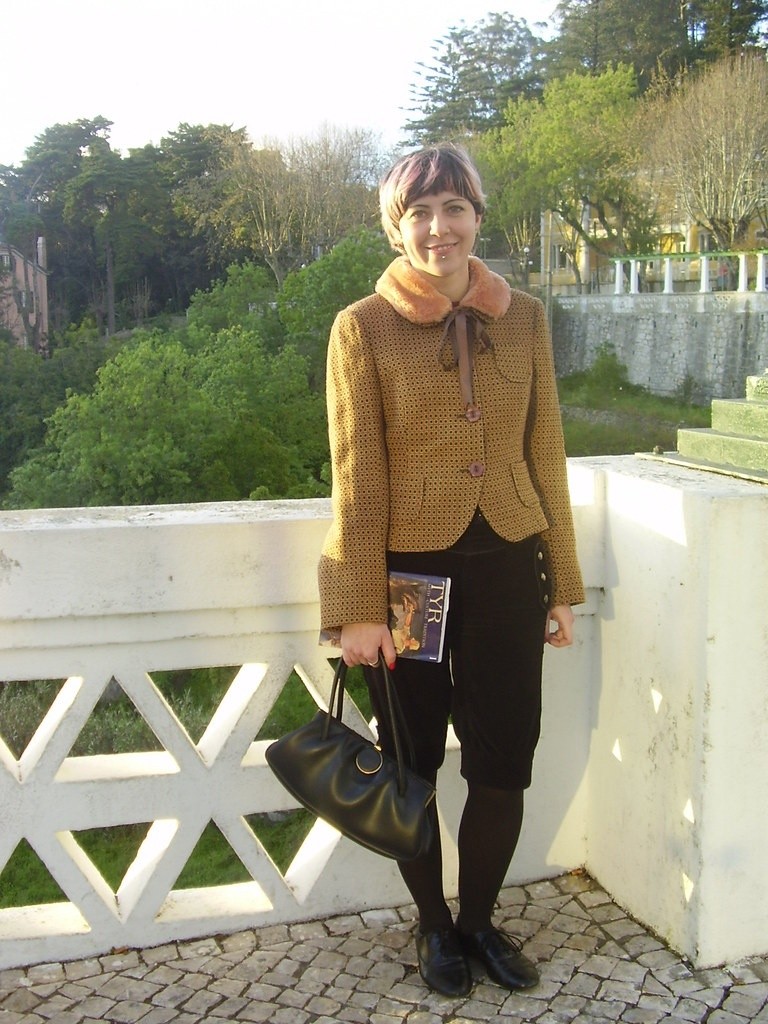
[454,913,540,990]
[415,920,473,997]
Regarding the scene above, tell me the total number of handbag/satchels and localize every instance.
[265,652,437,863]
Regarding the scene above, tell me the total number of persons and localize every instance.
[321,142,585,997]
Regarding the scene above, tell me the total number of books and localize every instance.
[317,570,451,664]
